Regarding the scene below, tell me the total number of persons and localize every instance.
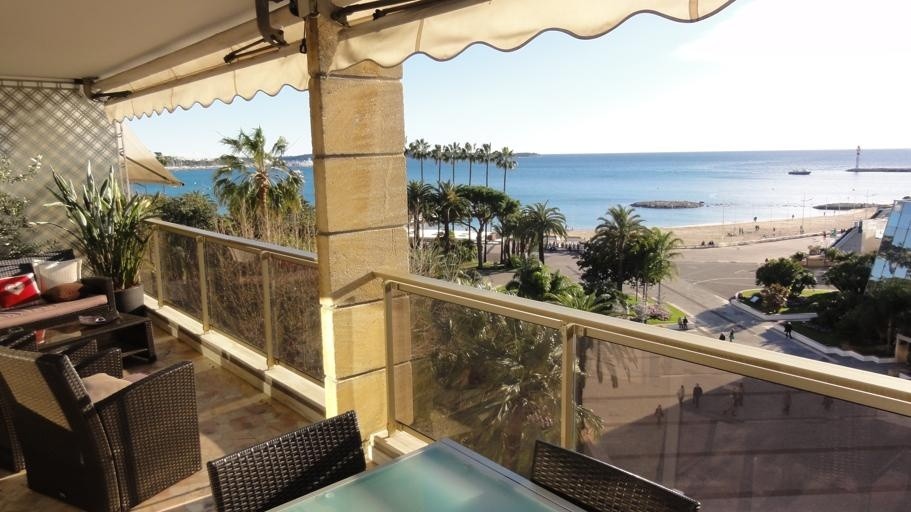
[678,316,684,328]
[653,404,664,425]
[677,384,686,408]
[732,387,739,408]
[784,321,793,339]
[782,391,793,415]
[720,332,726,341]
[722,391,736,417]
[729,327,737,343]
[739,382,745,406]
[693,382,703,409]
[684,316,689,329]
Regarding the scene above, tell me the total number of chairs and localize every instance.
[529,440,701,512]
[0,328,98,473]
[207,409,367,512]
[0,348,202,512]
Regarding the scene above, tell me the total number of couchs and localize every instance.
[0,249,116,337]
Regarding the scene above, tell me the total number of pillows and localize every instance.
[0,258,83,311]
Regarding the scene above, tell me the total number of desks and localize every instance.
[263,437,586,512]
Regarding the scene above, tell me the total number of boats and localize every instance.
[788,168,811,175]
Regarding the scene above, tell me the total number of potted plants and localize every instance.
[31,159,158,316]
